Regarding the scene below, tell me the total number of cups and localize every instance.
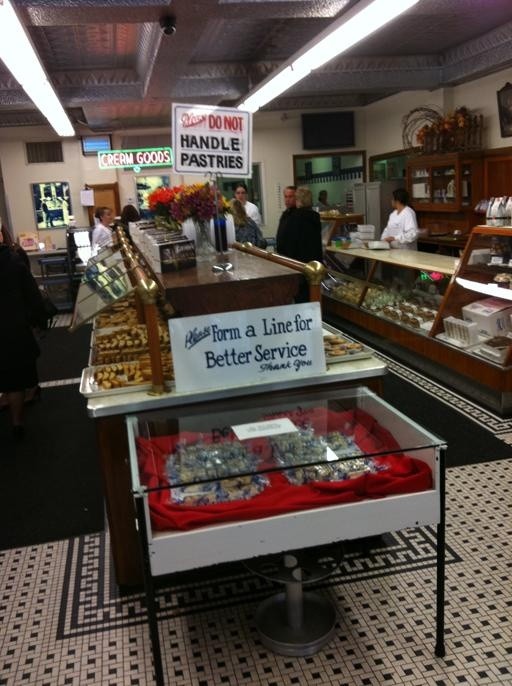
[328,209,336,217]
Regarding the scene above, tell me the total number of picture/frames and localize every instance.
[29,180,74,229]
[496,80,512,137]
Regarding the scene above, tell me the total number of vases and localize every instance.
[191,218,222,264]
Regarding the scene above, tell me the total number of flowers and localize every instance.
[147,179,223,225]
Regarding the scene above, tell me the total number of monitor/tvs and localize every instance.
[300,111,356,150]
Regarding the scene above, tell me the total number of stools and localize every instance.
[38,255,71,300]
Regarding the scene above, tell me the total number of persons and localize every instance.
[92,207,113,255]
[318,191,330,209]
[231,181,262,230]
[380,188,419,251]
[279,189,322,303]
[0,223,42,391]
[276,185,297,256]
[229,199,263,248]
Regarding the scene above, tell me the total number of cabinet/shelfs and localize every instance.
[67,225,390,591]
[128,390,446,684]
[404,153,478,212]
[322,224,512,421]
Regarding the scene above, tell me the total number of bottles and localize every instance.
[447,179,455,198]
